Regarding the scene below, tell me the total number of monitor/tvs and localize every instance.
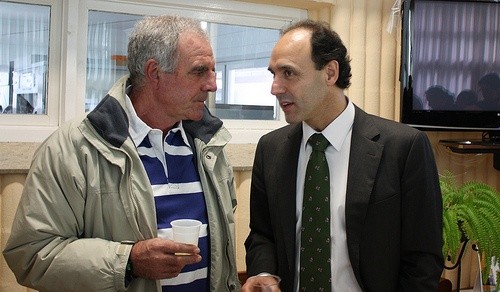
[400,0,500,134]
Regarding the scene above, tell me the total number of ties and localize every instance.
[300,133,333,292]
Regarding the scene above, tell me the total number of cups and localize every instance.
[170,218,202,264]
[247,275,281,292]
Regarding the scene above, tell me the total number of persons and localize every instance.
[424,72,500,111]
[241,18,447,292]
[2,14,242,292]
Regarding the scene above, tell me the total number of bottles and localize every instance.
[482,272,496,292]
